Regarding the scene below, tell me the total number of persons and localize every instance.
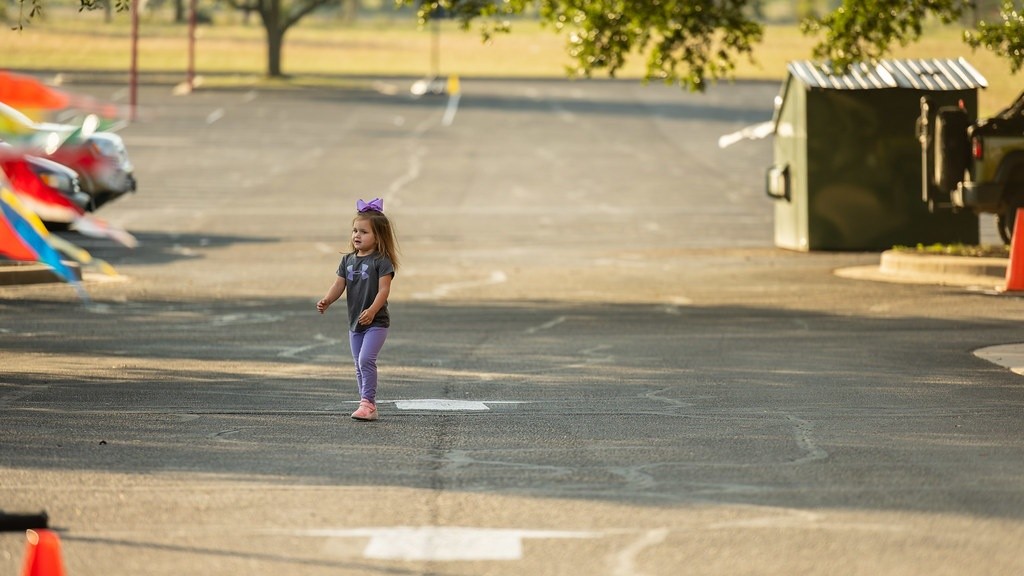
[317,199,401,420]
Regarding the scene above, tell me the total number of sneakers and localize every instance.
[351,399,378,421]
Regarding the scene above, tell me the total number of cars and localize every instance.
[1,99,140,206]
[0,138,95,230]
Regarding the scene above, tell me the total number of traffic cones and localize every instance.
[1000,204,1023,292]
[11,527,64,576]
